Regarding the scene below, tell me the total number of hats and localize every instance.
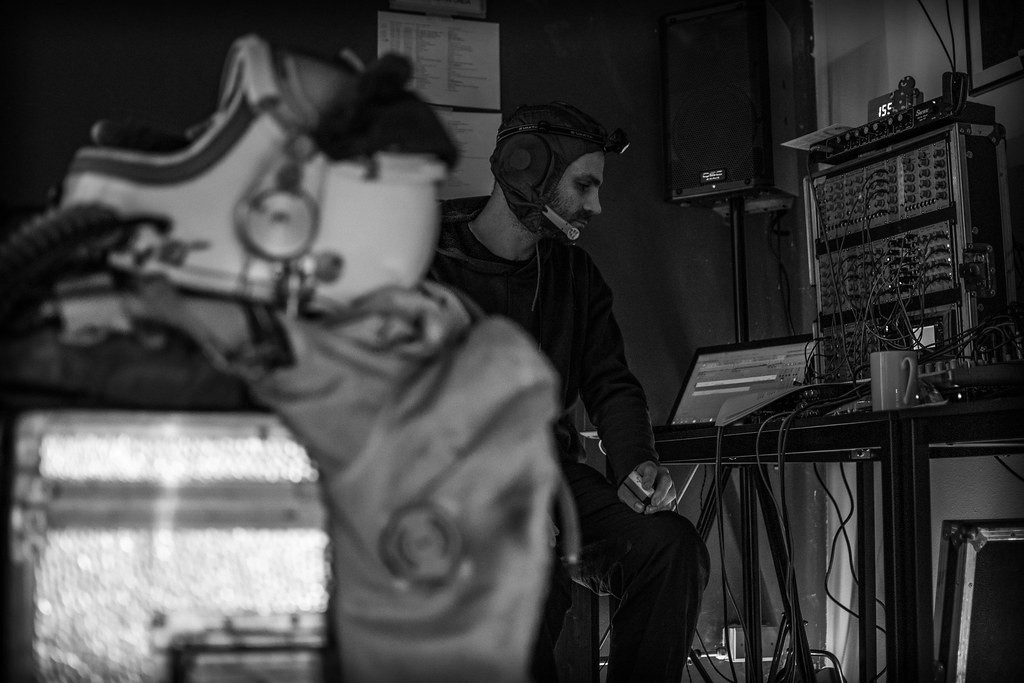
[492,101,611,244]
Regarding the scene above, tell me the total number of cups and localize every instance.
[869,349,919,411]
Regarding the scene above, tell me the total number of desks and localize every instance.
[598,406,1024,683]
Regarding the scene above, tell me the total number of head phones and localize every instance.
[497,102,608,204]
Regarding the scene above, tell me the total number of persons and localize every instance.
[422,102,710,683]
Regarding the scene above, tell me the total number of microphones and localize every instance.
[541,203,580,241]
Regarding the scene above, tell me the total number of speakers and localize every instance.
[659,1,801,217]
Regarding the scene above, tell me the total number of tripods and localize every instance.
[694,191,820,683]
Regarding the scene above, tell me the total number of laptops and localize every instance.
[577,333,817,441]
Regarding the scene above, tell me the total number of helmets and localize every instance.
[54,46,452,308]
